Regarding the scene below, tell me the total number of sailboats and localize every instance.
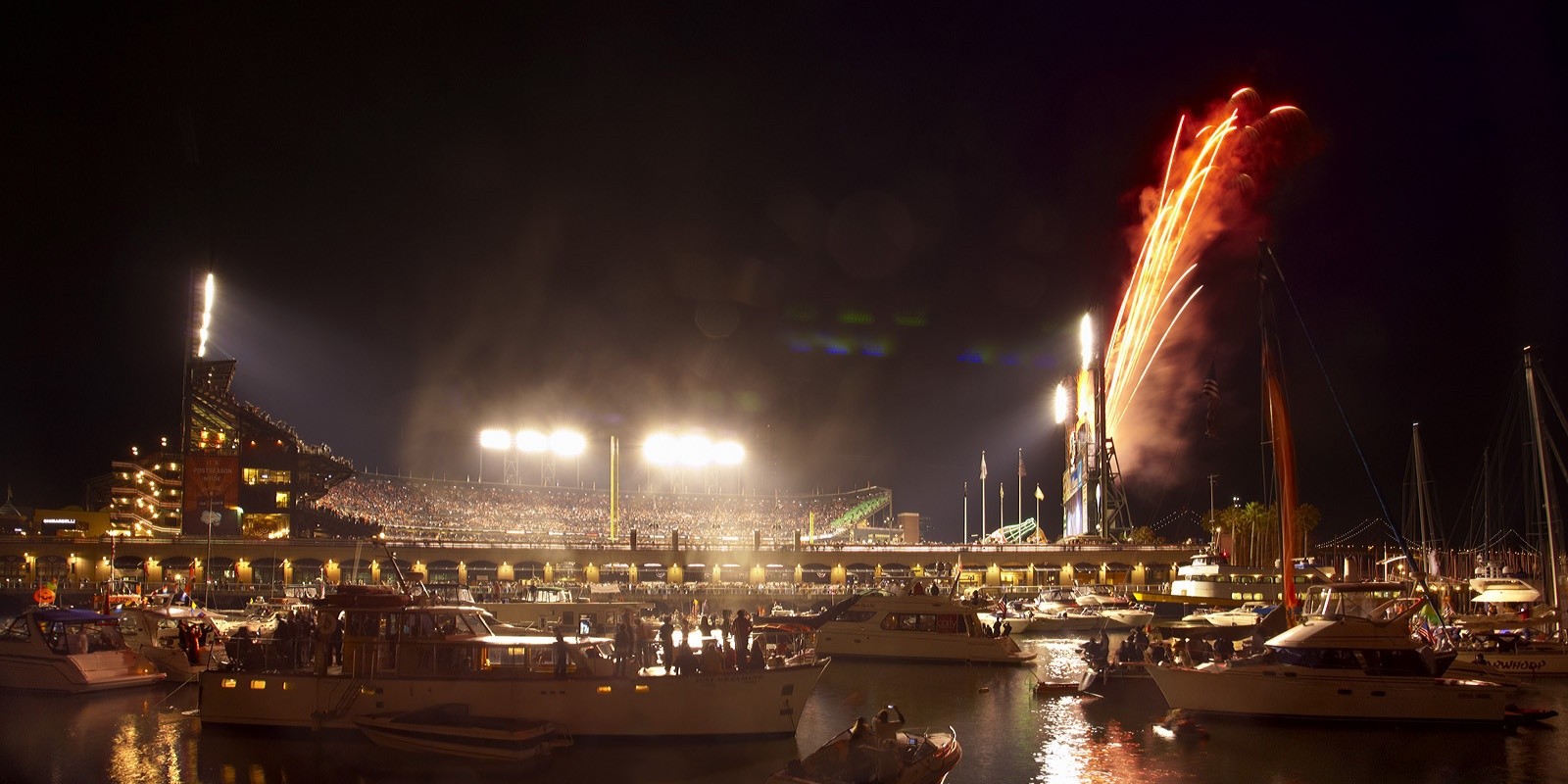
[0,221,1568,733]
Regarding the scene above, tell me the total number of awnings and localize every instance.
[248,558,284,568]
[200,557,234,567]
[157,557,195,567]
[110,556,144,566]
[339,558,785,571]
[289,559,325,568]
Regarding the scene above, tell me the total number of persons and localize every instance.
[77,627,88,654]
[552,635,568,674]
[100,634,110,651]
[273,610,345,669]
[614,609,754,675]
[1249,617,1264,654]
[854,703,905,742]
[493,581,500,603]
[176,619,213,663]
[985,618,1012,638]
[1466,603,1531,640]
[166,573,172,582]
[1079,625,1239,663]
[931,584,939,596]
[517,583,537,601]
[796,581,812,594]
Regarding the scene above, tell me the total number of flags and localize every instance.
[998,593,1007,619]
[181,592,188,601]
[191,601,198,615]
[1417,620,1435,645]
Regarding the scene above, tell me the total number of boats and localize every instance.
[765,717,964,784]
